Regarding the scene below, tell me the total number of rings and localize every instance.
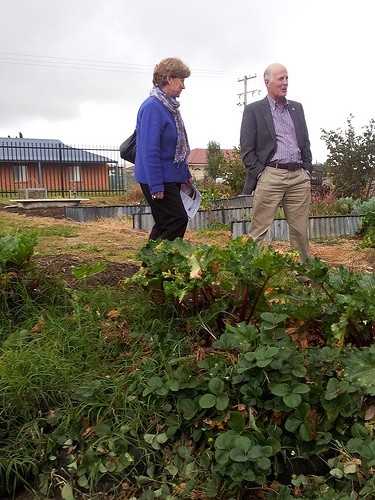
[153,196,156,198]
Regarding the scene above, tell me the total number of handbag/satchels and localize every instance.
[120,128,136,164]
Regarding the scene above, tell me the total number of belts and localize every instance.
[267,162,304,172]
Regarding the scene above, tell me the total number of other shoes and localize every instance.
[303,280,321,290]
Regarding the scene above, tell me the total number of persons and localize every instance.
[119,57,203,289]
[240,63,313,282]
